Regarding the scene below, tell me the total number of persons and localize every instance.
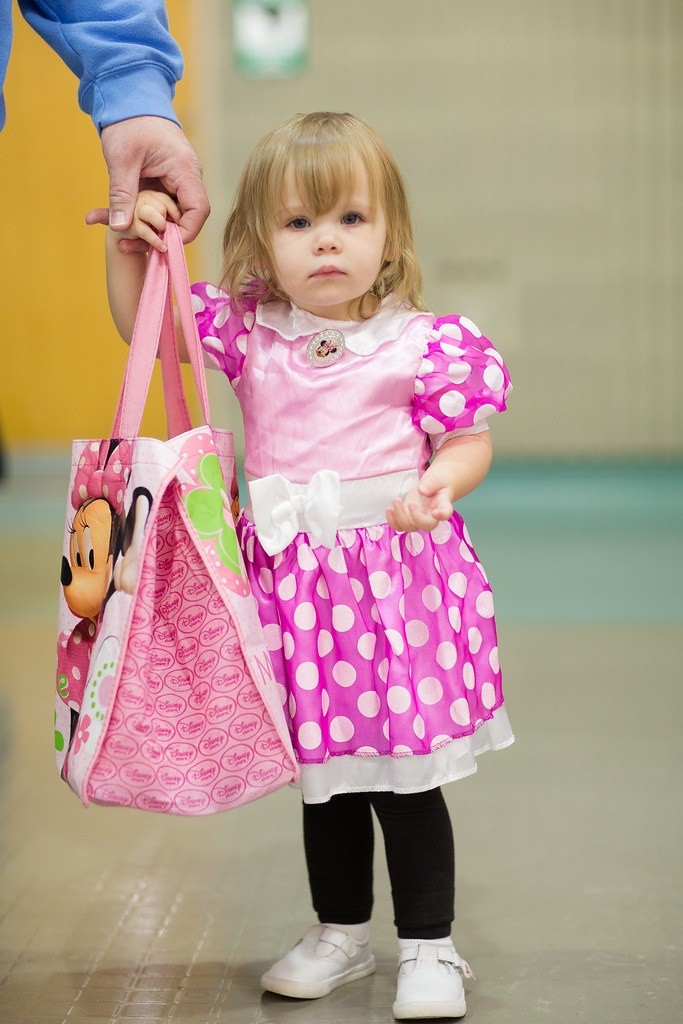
[104,110,514,1021]
[1,1,210,488]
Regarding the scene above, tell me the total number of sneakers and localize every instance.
[393,943,473,1019]
[260,925,376,998]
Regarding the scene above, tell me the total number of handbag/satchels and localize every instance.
[54,221,299,817]
